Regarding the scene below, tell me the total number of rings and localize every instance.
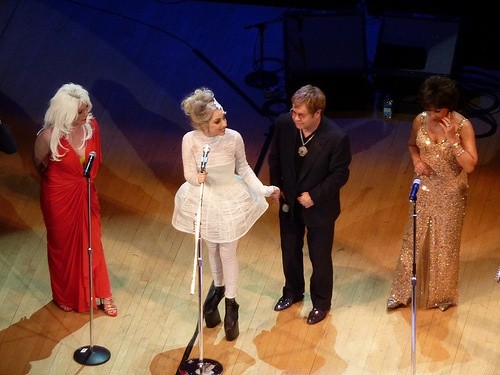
[417,173,420,176]
[445,123,450,127]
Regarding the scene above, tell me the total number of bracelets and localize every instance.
[450,142,465,158]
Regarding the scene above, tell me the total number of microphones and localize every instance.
[201,144,210,169]
[409,179,420,202]
[83,151,96,176]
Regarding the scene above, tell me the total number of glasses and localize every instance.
[289,107,313,120]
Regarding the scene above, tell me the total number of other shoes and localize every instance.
[203,279,225,328]
[386,296,400,308]
[53,299,73,312]
[439,304,450,311]
[223,297,239,342]
[100,297,117,316]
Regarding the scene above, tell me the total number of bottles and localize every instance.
[382,93,392,119]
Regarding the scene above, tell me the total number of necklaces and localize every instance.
[73,124,87,151]
[298,128,315,156]
[426,112,451,135]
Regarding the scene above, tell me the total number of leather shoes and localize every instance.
[273,295,304,311]
[307,306,331,324]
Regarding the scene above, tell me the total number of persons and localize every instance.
[171,86,280,341]
[267,84,352,326]
[387,75,477,311]
[32,82,118,317]
